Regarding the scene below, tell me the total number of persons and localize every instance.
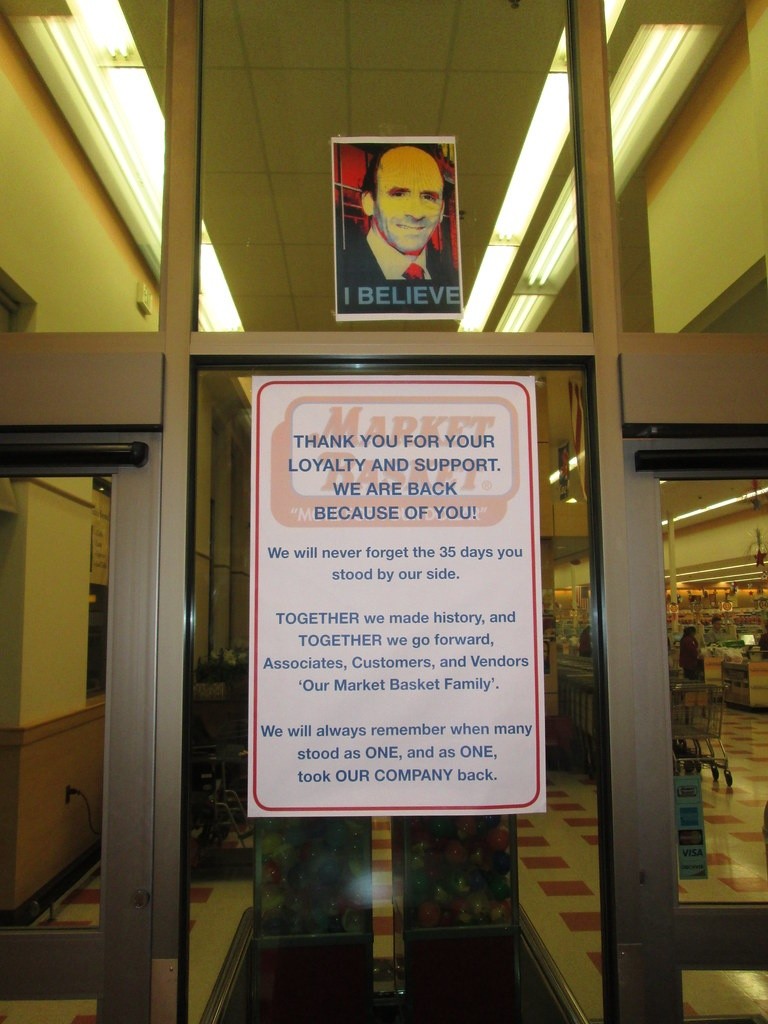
[704,617,723,646]
[343,144,459,313]
[679,627,699,681]
[758,619,768,660]
[543,641,550,674]
[578,625,594,657]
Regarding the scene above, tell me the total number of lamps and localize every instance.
[481,1,746,332]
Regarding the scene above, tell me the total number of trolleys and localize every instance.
[560,655,733,786]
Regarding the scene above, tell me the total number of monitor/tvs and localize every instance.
[741,635,755,645]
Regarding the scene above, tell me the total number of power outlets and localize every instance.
[65,785,71,805]
